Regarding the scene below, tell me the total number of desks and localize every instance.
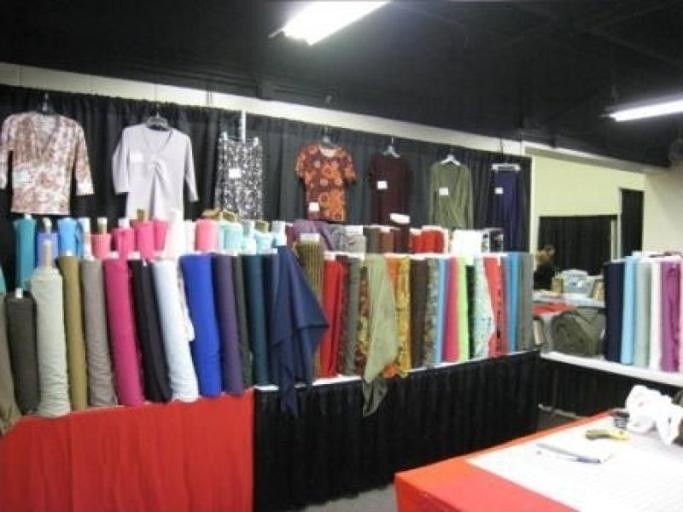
[394,409,682,512]
